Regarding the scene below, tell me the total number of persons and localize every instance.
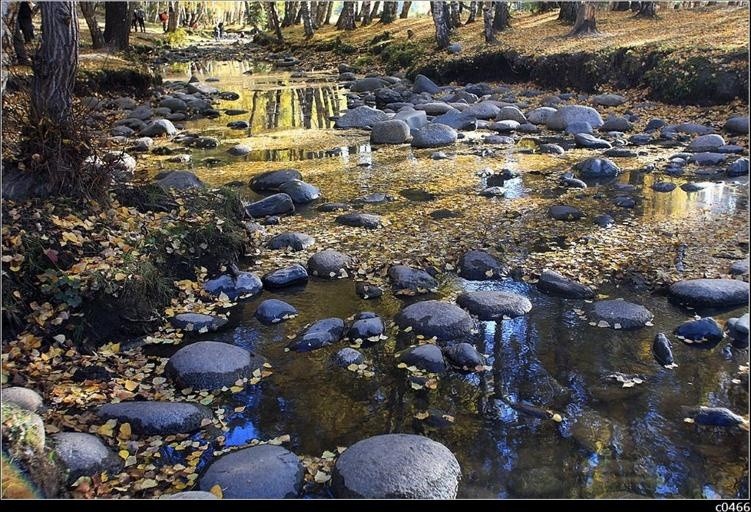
[130,5,201,34]
